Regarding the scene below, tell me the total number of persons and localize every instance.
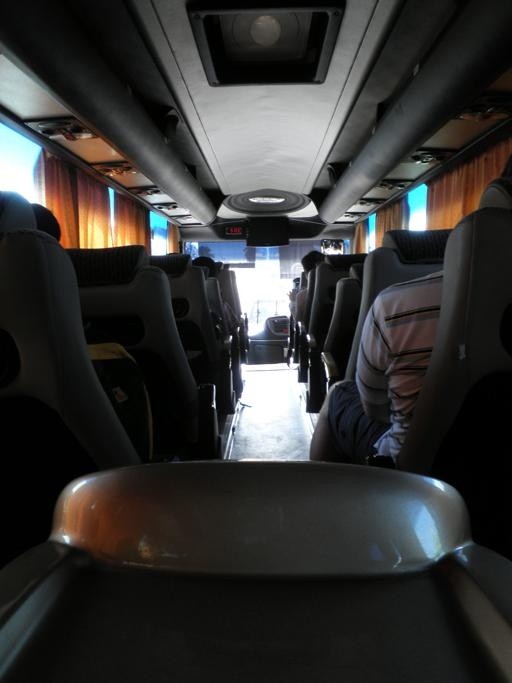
[31,202,60,240]
[309,267,444,461]
[192,256,240,326]
[287,250,326,320]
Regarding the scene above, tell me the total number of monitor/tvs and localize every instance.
[321,238,344,255]
